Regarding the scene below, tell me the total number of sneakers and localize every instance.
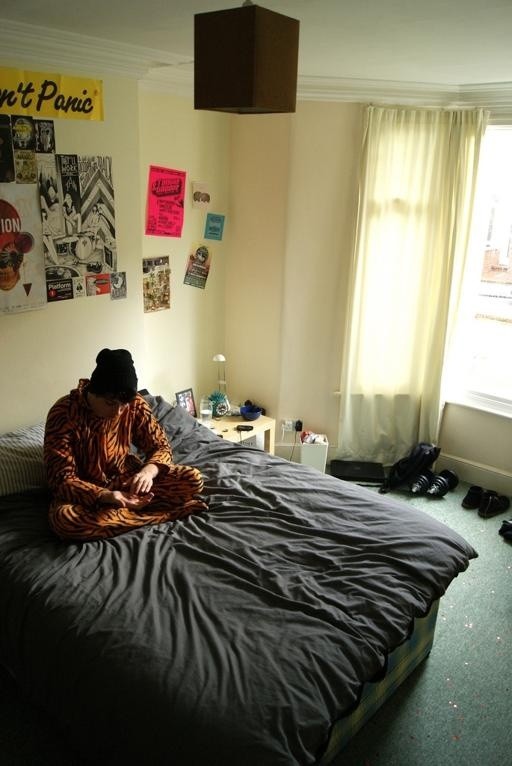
[462,485,499,509]
[478,494,510,518]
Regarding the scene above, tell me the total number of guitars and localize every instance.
[73,259,102,273]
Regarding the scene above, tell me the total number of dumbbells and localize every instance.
[412,469,434,494]
[427,468,458,499]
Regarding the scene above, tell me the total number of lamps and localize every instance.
[192,2,304,118]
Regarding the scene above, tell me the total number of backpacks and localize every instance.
[357,441,442,494]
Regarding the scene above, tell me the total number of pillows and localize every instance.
[1,423,49,495]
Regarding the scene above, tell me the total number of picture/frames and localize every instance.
[174,387,198,418]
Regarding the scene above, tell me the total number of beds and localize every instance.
[1,388,445,766]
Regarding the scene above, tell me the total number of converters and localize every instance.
[295,421,302,431]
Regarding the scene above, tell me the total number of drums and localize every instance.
[56,240,67,256]
[69,234,91,259]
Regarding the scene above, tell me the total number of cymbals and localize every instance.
[63,237,78,242]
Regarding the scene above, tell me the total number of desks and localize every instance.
[194,402,278,458]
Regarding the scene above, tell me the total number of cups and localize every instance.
[200,400,213,429]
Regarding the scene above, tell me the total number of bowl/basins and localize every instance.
[240,406,262,421]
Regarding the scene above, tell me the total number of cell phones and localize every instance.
[237,425,253,431]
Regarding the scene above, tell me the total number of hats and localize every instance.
[0,198,34,256]
[86,348,139,404]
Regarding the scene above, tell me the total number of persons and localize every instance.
[40,347,210,543]
